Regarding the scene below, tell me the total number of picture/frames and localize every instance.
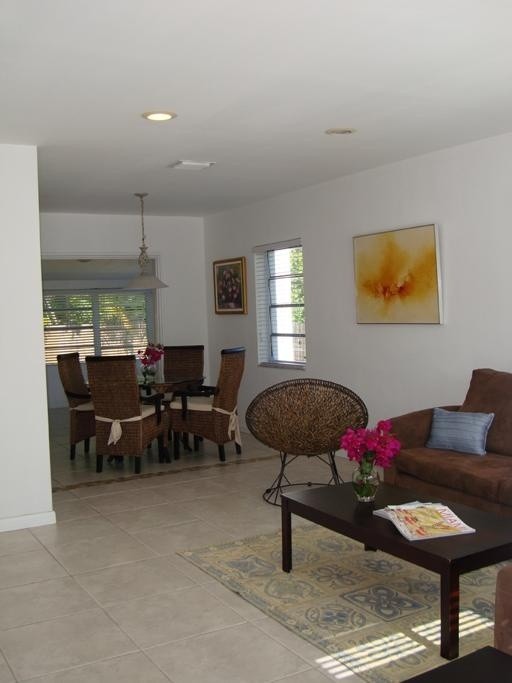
[352,223,445,325]
[213,256,248,314]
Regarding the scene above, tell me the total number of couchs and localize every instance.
[383,367,512,520]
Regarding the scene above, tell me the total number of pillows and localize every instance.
[425,407,495,456]
[459,369,512,457]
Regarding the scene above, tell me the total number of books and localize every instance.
[372,499,477,543]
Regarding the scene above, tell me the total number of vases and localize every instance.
[351,459,378,504]
[143,366,158,384]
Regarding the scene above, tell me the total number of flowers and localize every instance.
[340,419,401,470]
[135,342,166,366]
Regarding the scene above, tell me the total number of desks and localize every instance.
[395,646,512,682]
[139,375,204,394]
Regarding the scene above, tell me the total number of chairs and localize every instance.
[494,566,512,654]
[57,353,126,463]
[245,378,369,507]
[85,355,169,474]
[164,345,203,450]
[167,346,246,461]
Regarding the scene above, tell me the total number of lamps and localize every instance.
[126,192,168,290]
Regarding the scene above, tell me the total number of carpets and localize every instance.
[175,525,512,683]
